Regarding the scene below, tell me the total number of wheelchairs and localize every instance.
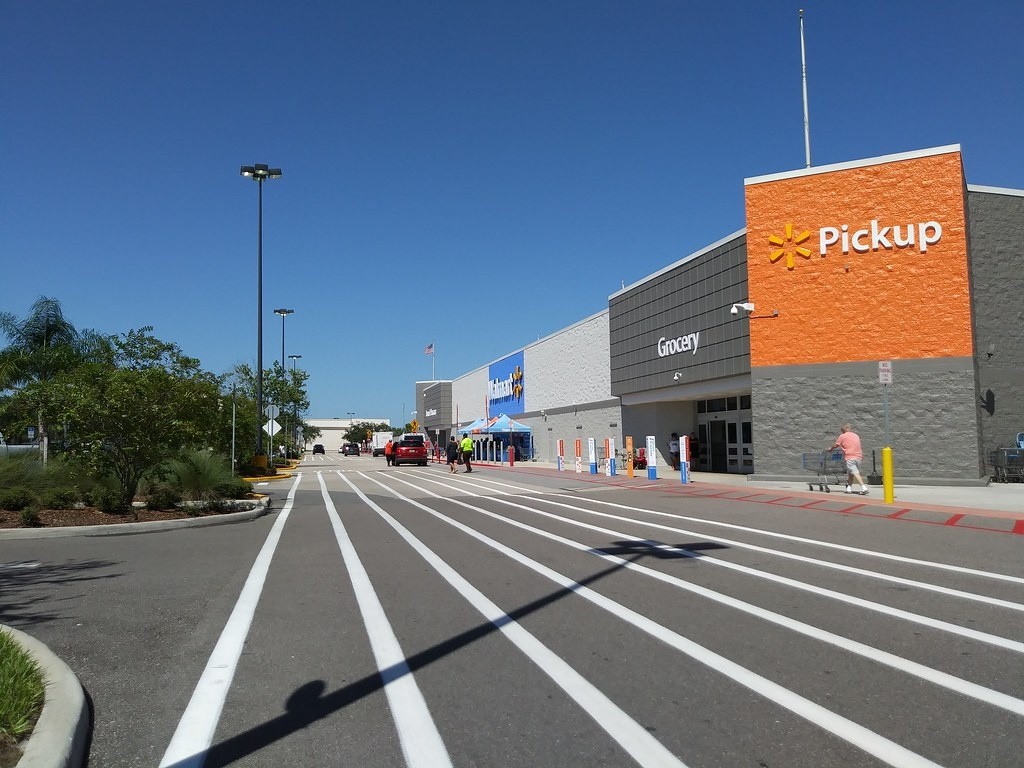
[627,448,647,470]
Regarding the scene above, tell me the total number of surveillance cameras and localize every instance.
[673,377,678,381]
[731,307,738,316]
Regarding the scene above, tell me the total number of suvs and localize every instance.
[392,440,428,466]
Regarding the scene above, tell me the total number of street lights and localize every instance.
[346,412,355,428]
[401,403,405,428]
[288,355,302,464]
[241,163,281,455]
[273,308,295,447]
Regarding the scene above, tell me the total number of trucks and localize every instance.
[372,432,394,456]
[400,433,425,443]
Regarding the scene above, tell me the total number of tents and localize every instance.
[455,414,532,465]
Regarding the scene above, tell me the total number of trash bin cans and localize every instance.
[506,446,515,462]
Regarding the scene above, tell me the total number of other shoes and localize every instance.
[859,489,869,495]
[453,470,458,473]
[463,469,472,473]
[844,490,851,493]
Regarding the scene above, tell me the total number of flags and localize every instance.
[425,343,433,354]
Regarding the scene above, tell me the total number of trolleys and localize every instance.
[518,448,538,462]
[803,449,849,493]
[990,444,1024,483]
[598,446,618,469]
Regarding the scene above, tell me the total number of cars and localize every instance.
[312,444,325,454]
[345,444,360,456]
[338,447,343,452]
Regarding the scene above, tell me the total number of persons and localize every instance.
[424,439,476,462]
[385,440,393,467]
[825,423,870,495]
[459,433,474,473]
[446,436,458,473]
[690,432,700,471]
[669,432,679,470]
